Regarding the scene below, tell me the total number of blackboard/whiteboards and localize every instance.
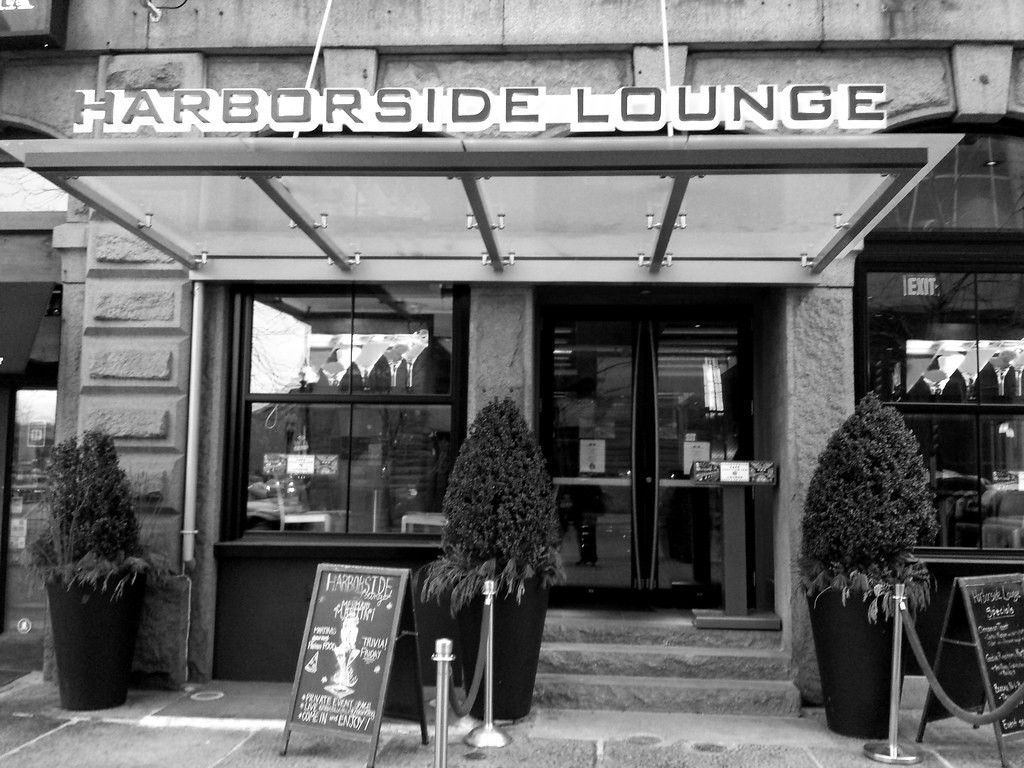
[918,573,1024,742]
[287,563,425,739]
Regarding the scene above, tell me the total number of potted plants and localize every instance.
[27,431,156,713]
[443,396,563,721]
[796,392,942,741]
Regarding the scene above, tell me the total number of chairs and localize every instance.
[401,511,453,535]
[265,451,335,534]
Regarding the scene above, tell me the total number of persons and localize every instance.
[571,486,609,567]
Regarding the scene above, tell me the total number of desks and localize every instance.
[689,458,782,630]
